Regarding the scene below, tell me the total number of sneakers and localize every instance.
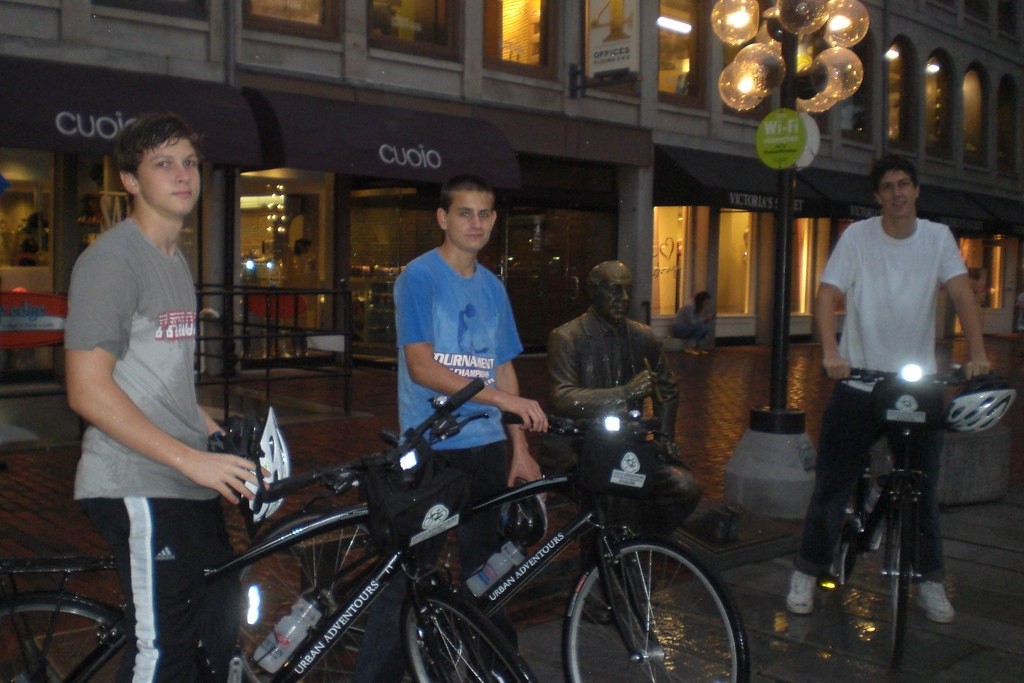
[915,580,956,622]
[786,569,818,614]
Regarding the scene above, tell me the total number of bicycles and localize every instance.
[232,372,751,683]
[827,360,980,675]
[1,372,541,683]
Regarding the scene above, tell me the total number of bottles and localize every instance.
[467,539,530,596]
[866,474,891,512]
[253,591,328,674]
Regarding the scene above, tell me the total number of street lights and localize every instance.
[708,0,868,522]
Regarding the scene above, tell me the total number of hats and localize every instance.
[21,212,49,235]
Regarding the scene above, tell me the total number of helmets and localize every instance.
[495,485,550,549]
[944,378,1016,434]
[234,404,291,526]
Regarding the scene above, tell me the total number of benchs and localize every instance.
[277,496,576,634]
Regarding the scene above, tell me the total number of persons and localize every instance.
[542,261,703,625]
[352,173,549,683]
[64,111,271,683]
[787,154,993,623]
[670,290,716,355]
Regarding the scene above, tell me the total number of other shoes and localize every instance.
[684,347,700,354]
[695,347,709,354]
